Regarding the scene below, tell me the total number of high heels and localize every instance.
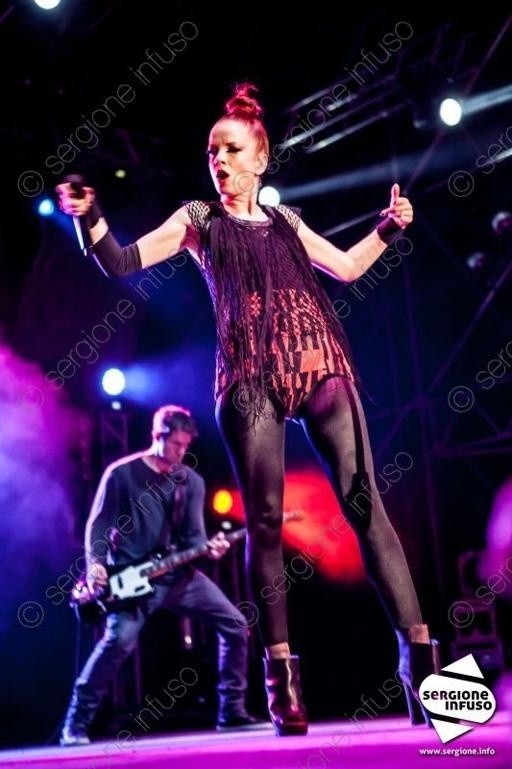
[399,638,459,727]
[265,655,308,735]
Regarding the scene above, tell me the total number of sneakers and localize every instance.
[60,726,91,745]
[216,714,273,731]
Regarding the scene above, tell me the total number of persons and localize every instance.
[55,405,274,749]
[51,79,461,734]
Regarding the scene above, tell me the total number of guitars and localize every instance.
[69,508,304,624]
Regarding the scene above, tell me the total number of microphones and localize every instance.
[67,174,94,257]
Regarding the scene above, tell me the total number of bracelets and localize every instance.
[376,214,407,252]
[84,203,102,229]
